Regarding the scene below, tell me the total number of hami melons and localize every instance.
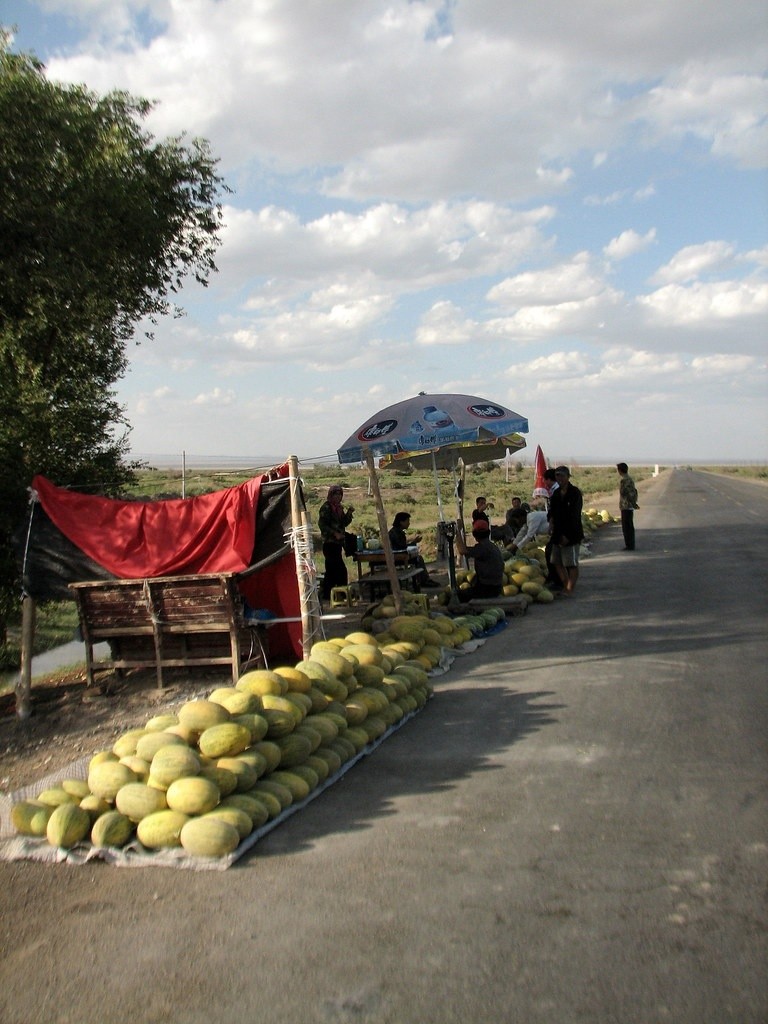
[11,590,505,859]
[437,508,620,603]
[367,539,381,549]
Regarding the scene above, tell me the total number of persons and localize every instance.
[456,519,505,603]
[388,512,441,587]
[544,466,586,599]
[318,485,355,600]
[472,497,549,549]
[617,463,640,551]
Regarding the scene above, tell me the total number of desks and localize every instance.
[355,544,418,591]
[358,567,424,601]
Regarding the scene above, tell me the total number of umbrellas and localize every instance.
[337,391,529,585]
[378,433,527,566]
[533,444,553,512]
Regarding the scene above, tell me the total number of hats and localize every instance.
[555,466,569,478]
[473,519,488,530]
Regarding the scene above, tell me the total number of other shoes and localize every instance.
[622,548,635,550]
[556,589,574,598]
[420,580,440,588]
[548,583,564,591]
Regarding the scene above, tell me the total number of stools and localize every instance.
[329,586,355,609]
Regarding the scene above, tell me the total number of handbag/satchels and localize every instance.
[343,532,357,557]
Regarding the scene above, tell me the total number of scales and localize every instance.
[439,521,528,617]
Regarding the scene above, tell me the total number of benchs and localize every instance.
[66,571,264,689]
